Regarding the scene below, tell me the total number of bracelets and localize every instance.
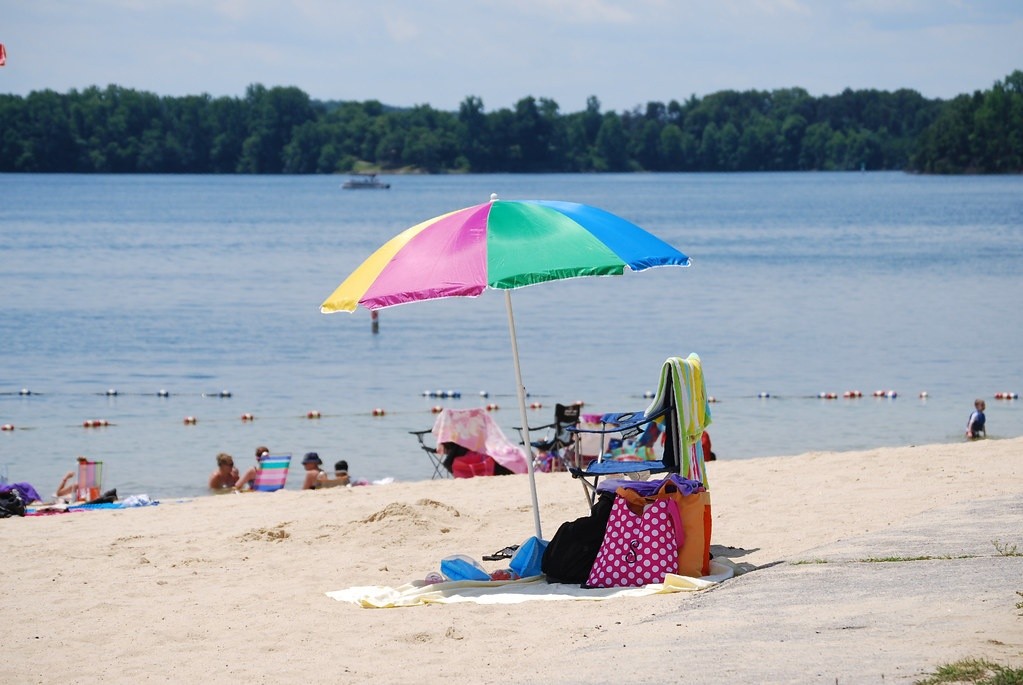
[63,479,66,481]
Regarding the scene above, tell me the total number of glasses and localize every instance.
[227,463,233,467]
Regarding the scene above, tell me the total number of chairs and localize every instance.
[559,348,713,512]
[408,406,486,478]
[511,402,582,472]
[76,460,102,500]
[239,452,292,492]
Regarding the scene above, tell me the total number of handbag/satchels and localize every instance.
[643,481,712,577]
[542,490,617,584]
[585,487,685,588]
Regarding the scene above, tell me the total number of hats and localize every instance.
[301,452,322,464]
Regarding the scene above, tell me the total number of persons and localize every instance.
[234,447,270,488]
[335,461,348,476]
[209,453,239,488]
[56,457,87,497]
[965,399,986,438]
[301,452,325,490]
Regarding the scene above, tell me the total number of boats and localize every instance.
[341,173,390,189]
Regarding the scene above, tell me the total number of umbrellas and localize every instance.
[320,192,692,540]
